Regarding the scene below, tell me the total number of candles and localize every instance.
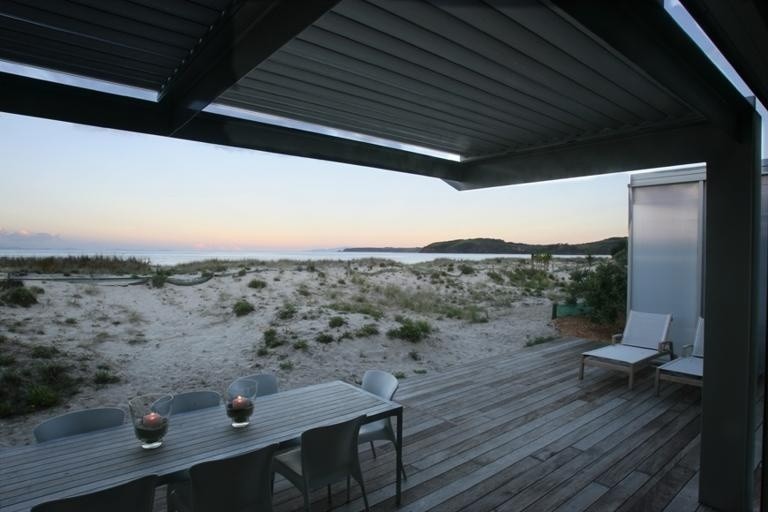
[142,411,162,426]
[233,394,248,408]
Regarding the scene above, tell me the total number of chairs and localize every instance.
[228,374,301,449]
[654,315,703,396]
[169,441,274,508]
[356,371,408,482]
[274,412,369,512]
[580,309,674,392]
[32,474,157,512]
[32,408,126,442]
[152,390,222,491]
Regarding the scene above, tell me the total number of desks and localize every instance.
[0,378,404,510]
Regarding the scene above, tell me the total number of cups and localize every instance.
[222,375,260,428]
[128,390,172,450]
